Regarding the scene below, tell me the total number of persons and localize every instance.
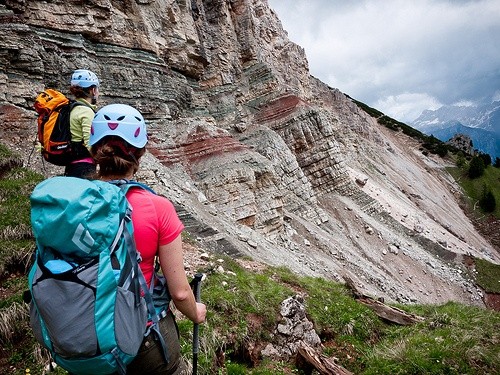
[64,70,100,181]
[66,104,206,375]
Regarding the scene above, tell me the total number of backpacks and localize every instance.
[34,89,97,166]
[24,176,169,375]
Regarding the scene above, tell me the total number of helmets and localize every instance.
[70,69,100,89]
[90,103,147,149]
